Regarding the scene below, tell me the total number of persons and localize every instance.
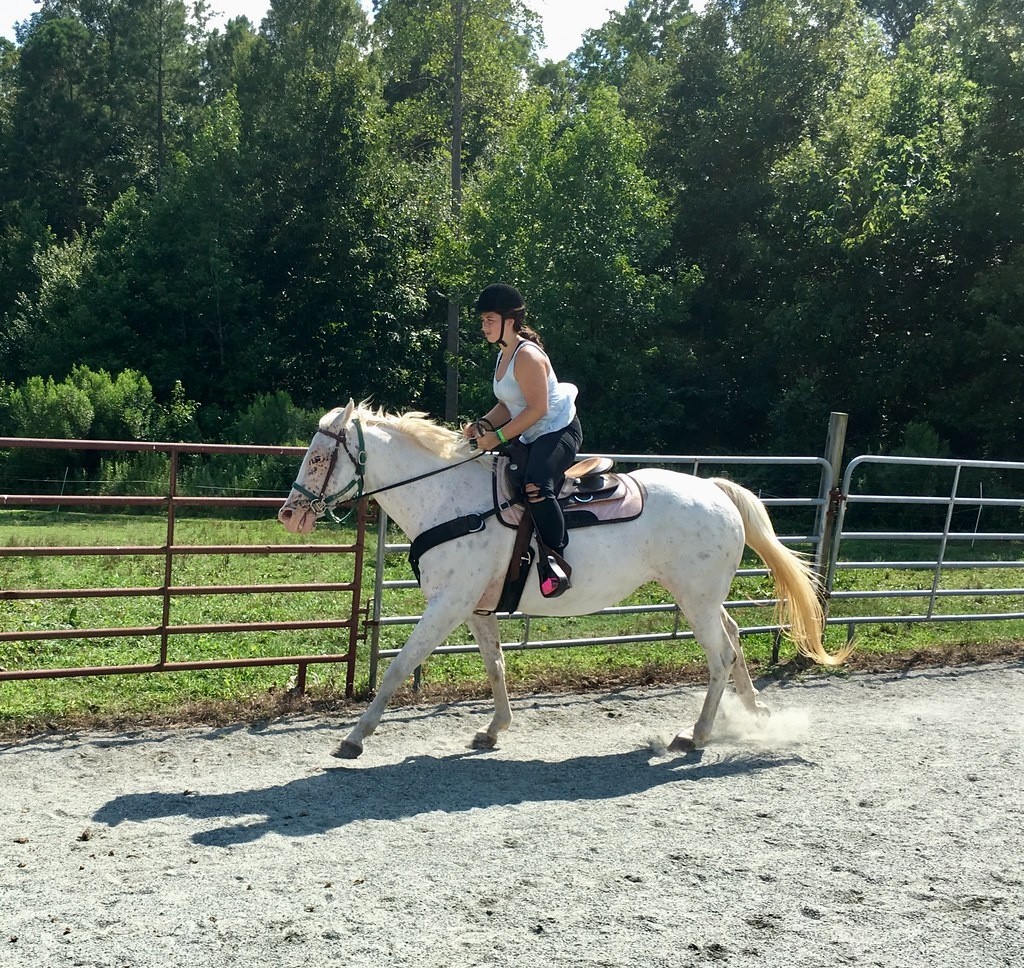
[465,284,583,595]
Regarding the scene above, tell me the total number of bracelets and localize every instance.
[496,429,508,443]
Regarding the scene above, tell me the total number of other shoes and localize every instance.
[540,577,559,594]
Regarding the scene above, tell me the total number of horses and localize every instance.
[278,394,864,765]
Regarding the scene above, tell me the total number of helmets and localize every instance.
[476,284,524,316]
